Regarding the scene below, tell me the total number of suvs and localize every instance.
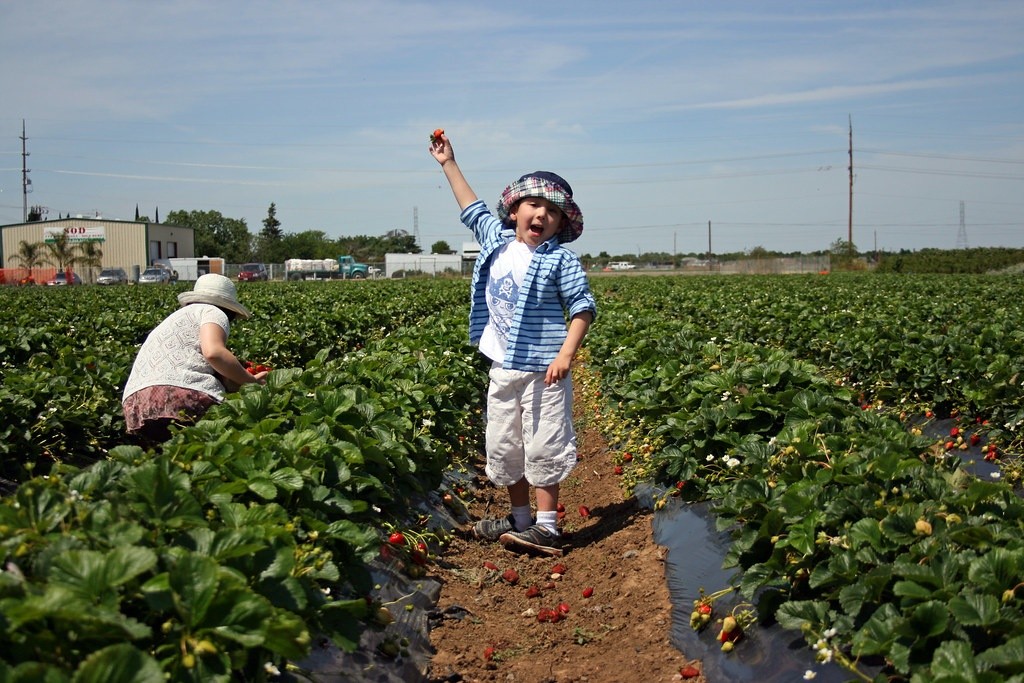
[95,267,129,285]
[237,263,269,282]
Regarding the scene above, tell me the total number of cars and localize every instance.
[47,272,82,286]
[139,267,171,284]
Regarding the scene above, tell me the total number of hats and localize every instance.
[177,273,251,319]
[496,171,583,245]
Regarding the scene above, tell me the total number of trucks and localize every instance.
[284,255,369,281]
[609,262,636,270]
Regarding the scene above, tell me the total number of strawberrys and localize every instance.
[243,361,272,376]
[853,392,1020,483]
[433,128,444,140]
[377,389,743,679]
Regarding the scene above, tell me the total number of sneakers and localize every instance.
[473,513,534,542]
[500,525,563,556]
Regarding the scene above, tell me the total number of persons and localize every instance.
[122,273,268,445]
[429,128,596,555]
